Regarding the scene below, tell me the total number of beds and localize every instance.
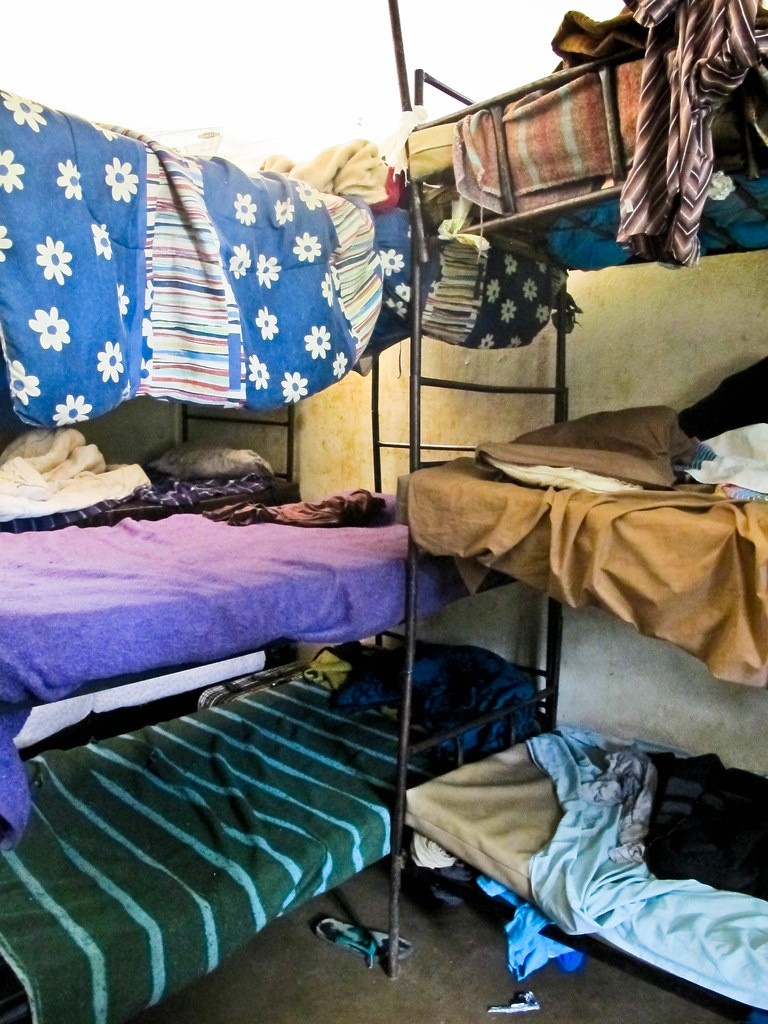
[0,0,768,1024]
